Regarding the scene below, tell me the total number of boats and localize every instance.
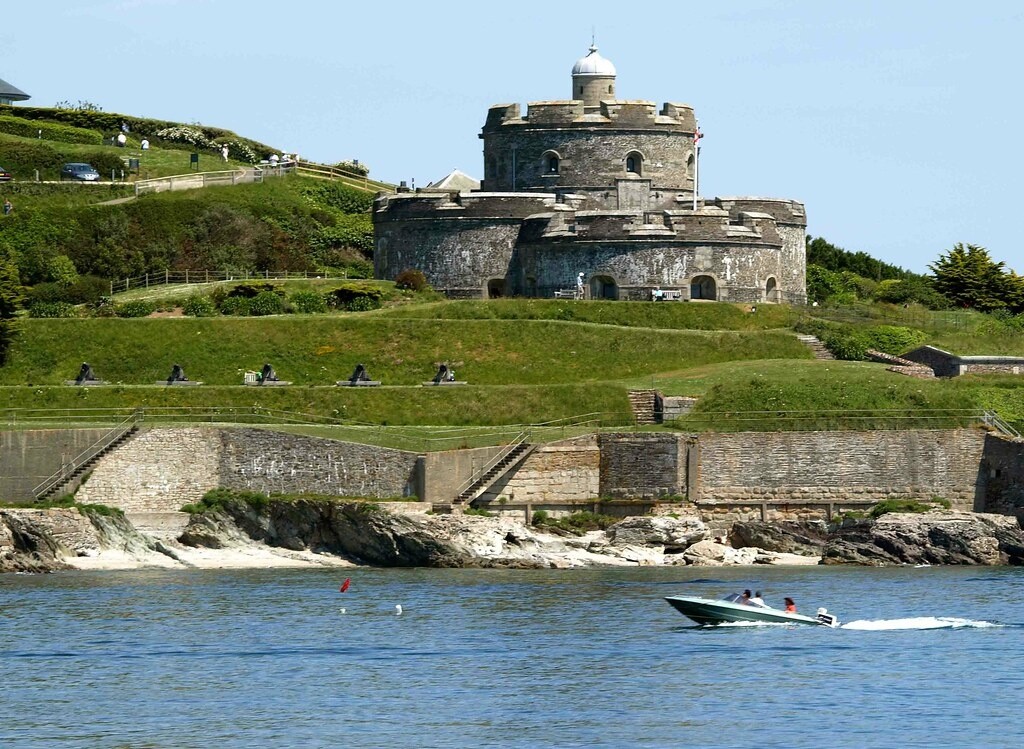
[661,590,842,630]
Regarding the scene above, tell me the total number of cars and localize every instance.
[0,166,13,181]
[59,163,101,182]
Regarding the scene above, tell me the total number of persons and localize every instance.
[142,136,151,151]
[743,586,765,609]
[221,143,229,162]
[784,596,797,615]
[118,129,126,145]
[653,286,667,303]
[269,151,298,175]
[2,198,13,214]
[577,273,586,299]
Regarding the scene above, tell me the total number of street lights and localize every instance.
[510,142,519,192]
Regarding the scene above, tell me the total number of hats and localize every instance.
[784,598,794,605]
[756,591,761,595]
